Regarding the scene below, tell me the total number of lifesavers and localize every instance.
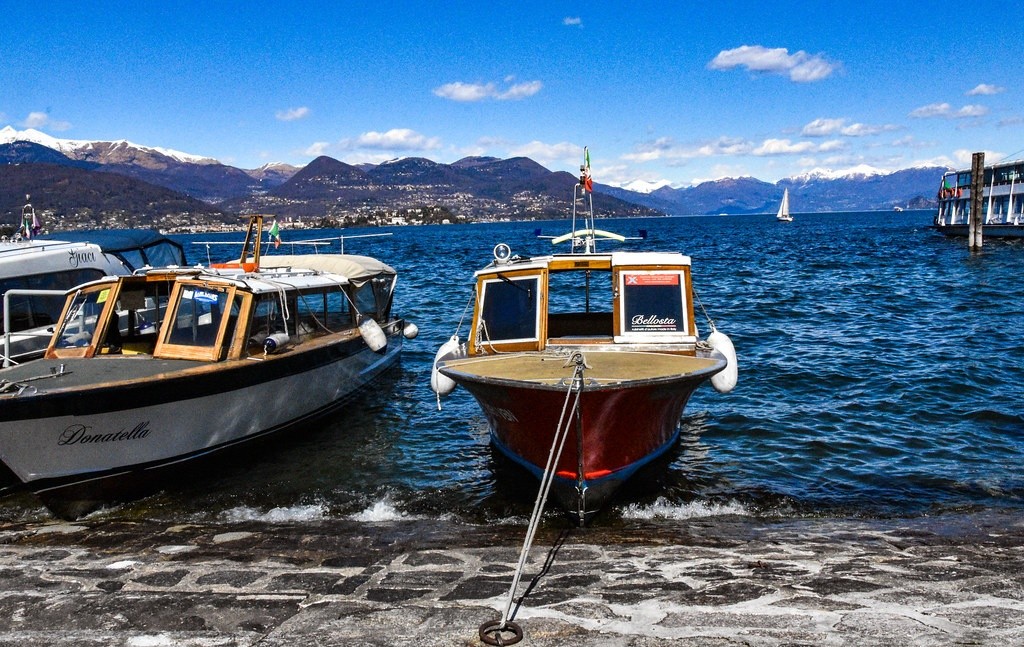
[209,262,260,273]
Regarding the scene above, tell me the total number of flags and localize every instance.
[581,145,594,191]
[269,220,281,250]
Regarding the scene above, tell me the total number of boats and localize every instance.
[1,241,132,362]
[933,160,1024,240]
[0,256,403,520]
[434,254,742,524]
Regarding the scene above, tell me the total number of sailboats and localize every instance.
[776,188,797,223]
